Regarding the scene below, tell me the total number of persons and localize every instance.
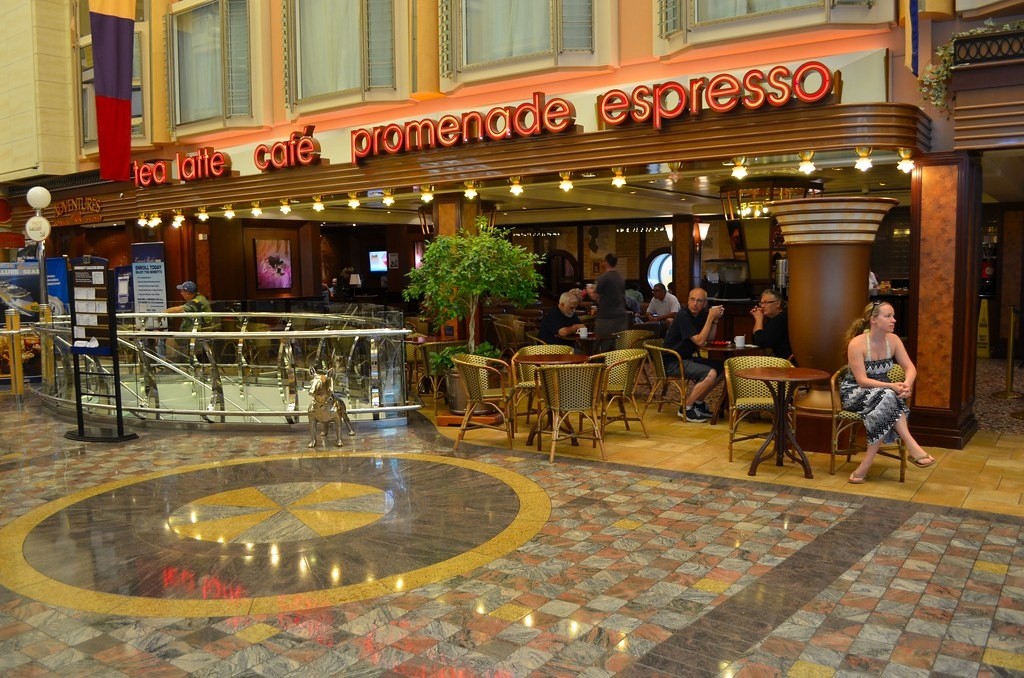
[570,280,681,335]
[329,278,339,299]
[661,288,724,422]
[536,291,586,356]
[752,289,796,366]
[587,253,626,363]
[839,301,934,482]
[162,282,213,364]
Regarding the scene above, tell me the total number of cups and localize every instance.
[710,306,719,309]
[586,284,593,289]
[577,327,588,337]
[733,336,746,348]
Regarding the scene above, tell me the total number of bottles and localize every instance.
[591,306,597,316]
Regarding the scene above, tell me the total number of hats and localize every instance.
[177,281,196,291]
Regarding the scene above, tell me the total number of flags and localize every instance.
[89,0,136,180]
[904,0,918,76]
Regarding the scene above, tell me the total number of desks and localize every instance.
[575,314,598,332]
[734,367,831,480]
[698,343,773,425]
[510,308,544,334]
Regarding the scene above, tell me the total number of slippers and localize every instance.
[907,453,935,467]
[848,472,866,484]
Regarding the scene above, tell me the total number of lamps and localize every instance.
[138,146,915,243]
[350,274,361,297]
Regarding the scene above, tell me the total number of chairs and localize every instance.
[723,356,799,463]
[112,312,687,463]
[511,343,575,432]
[831,363,916,486]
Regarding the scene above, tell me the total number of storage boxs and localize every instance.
[703,259,749,286]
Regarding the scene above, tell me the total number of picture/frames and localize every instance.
[253,235,294,291]
[414,240,433,272]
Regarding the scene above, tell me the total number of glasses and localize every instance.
[651,289,663,293]
[871,300,883,315]
[758,301,777,306]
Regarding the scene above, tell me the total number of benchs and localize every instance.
[554,332,615,356]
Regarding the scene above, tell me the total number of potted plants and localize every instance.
[400,215,546,415]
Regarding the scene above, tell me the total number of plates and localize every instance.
[710,341,731,345]
[574,331,594,334]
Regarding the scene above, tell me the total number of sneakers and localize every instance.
[677,406,707,423]
[693,401,713,417]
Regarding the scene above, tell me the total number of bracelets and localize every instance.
[712,321,718,325]
